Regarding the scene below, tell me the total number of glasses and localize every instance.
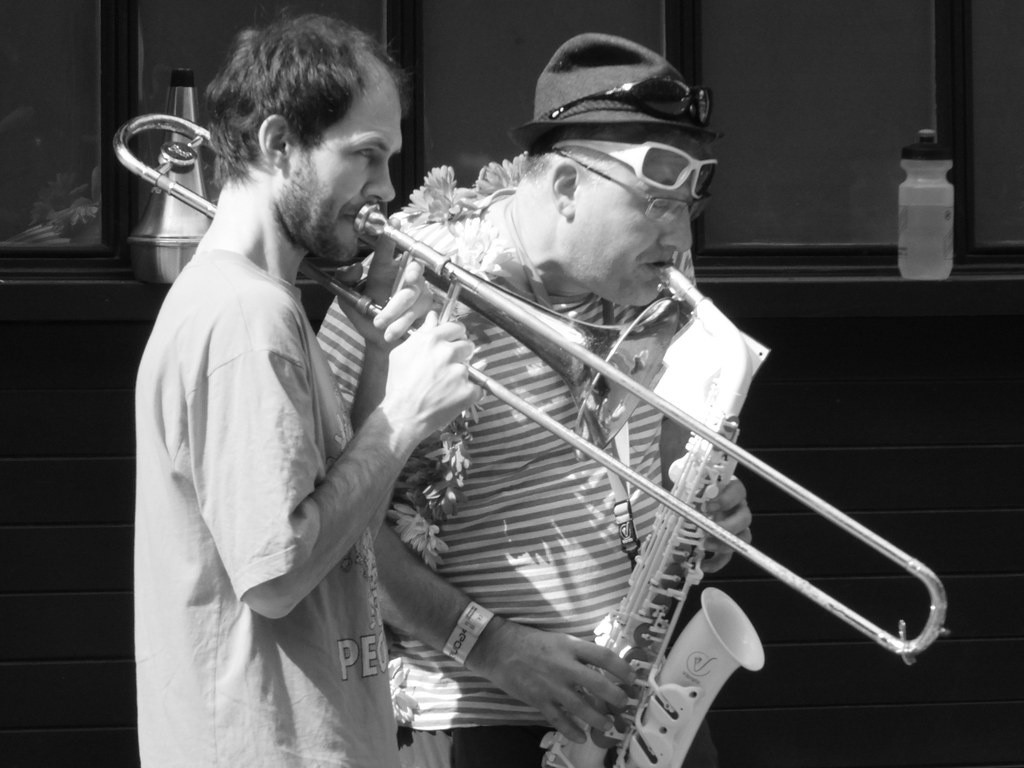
[549,78,712,126]
[553,139,718,199]
[554,147,712,221]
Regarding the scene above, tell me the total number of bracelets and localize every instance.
[442,601,494,665]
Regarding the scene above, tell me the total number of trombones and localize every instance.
[112,110,951,670]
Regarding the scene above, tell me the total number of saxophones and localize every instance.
[535,264,766,768]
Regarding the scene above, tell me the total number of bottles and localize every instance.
[897,129,954,280]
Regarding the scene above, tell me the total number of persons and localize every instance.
[132,14,486,768]
[315,33,754,768]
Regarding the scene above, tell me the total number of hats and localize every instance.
[508,33,724,151]
